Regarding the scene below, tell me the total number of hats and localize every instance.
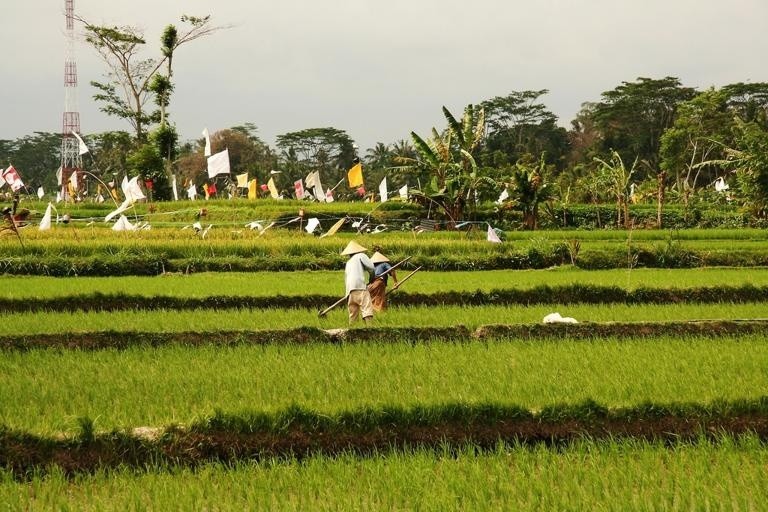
[369,251,390,263]
[338,239,368,256]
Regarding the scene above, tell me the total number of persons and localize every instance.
[368,250,400,307]
[341,240,376,328]
